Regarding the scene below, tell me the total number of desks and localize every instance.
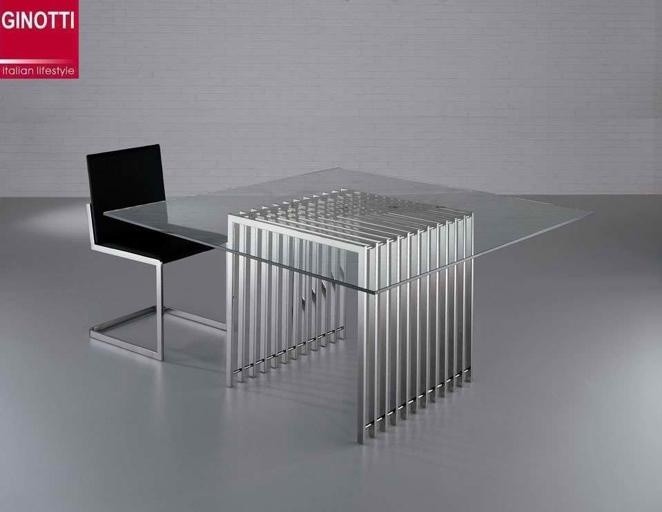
[99,166,595,446]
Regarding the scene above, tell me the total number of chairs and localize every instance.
[81,140,227,364]
